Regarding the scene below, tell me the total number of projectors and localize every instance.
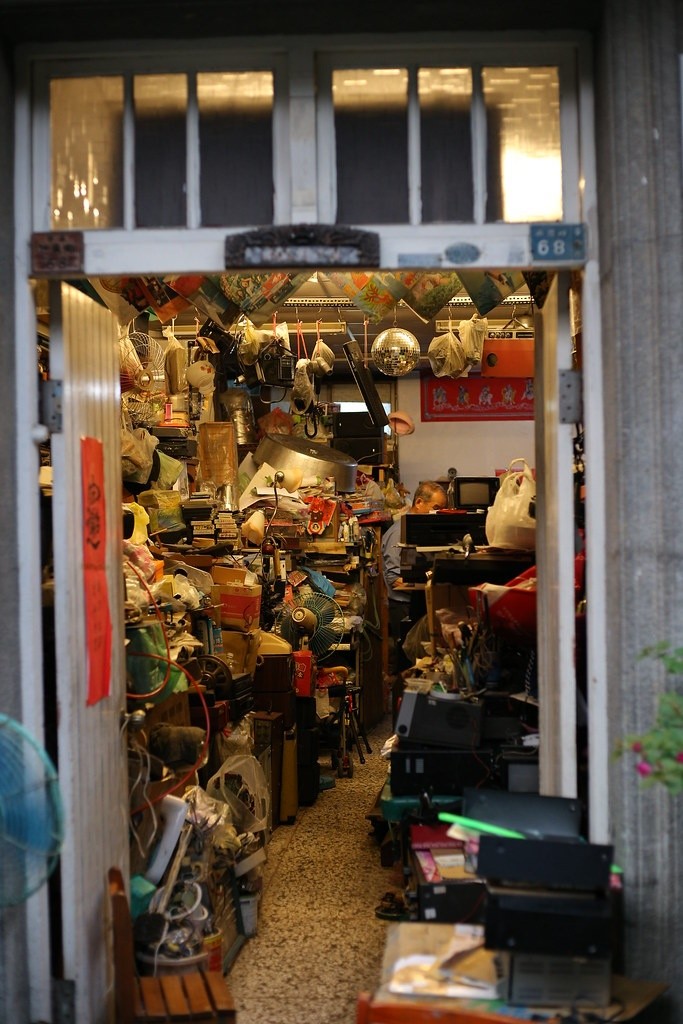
[394,692,487,747]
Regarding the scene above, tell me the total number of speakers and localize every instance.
[330,412,387,465]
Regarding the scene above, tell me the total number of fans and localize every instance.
[274,592,345,664]
[118,331,171,419]
[0,711,65,910]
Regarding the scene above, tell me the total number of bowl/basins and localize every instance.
[252,433,358,493]
[186,361,214,389]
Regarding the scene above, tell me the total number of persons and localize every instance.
[382,480,448,734]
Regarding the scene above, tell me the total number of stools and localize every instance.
[377,778,463,821]
[331,687,372,765]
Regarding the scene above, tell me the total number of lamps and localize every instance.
[436,319,535,331]
[265,468,303,536]
[163,322,346,336]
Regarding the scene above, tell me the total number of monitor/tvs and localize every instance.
[454,476,500,512]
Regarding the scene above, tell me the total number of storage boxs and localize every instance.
[511,955,611,1009]
[210,584,262,633]
[409,850,624,974]
[255,694,316,730]
[253,655,292,692]
[216,630,261,675]
[150,426,188,438]
[133,690,190,763]
[331,412,382,465]
[399,514,489,547]
[212,566,255,586]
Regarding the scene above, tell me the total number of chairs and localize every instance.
[106,865,236,1024]
